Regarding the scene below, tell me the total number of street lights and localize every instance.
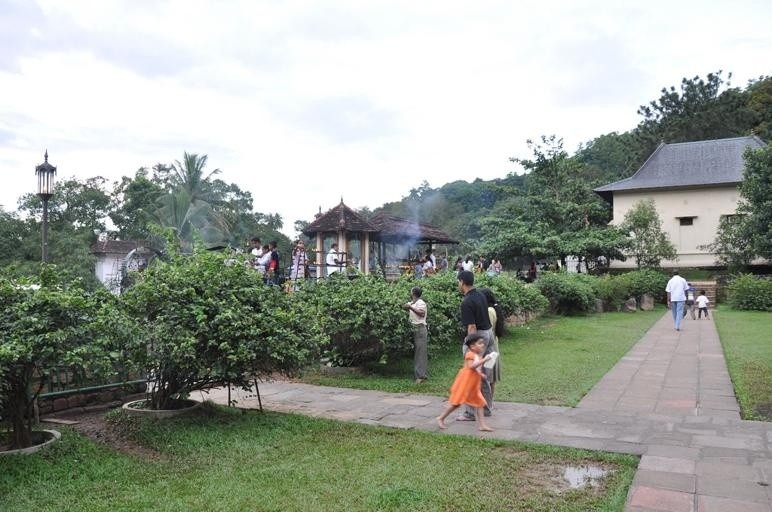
[35,148,56,264]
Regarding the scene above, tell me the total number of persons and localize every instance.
[358,250,374,273]
[326,243,340,277]
[414,250,448,280]
[288,240,310,281]
[695,290,713,320]
[483,288,502,408]
[437,334,494,431]
[247,237,280,285]
[683,284,697,320]
[404,286,430,384]
[665,269,690,328]
[453,254,502,277]
[515,260,561,284]
[456,268,493,420]
